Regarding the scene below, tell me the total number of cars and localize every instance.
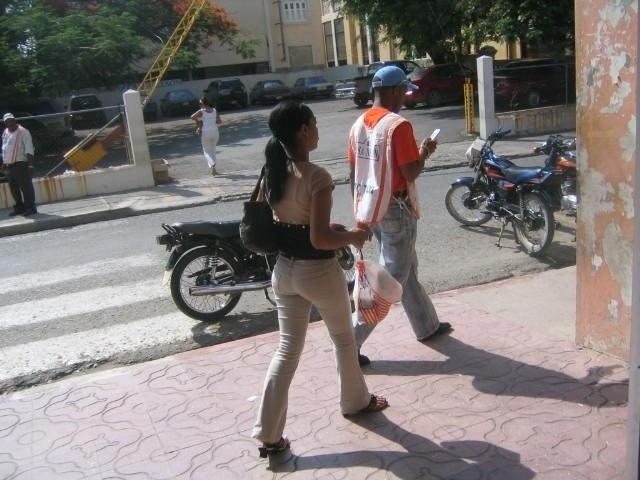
[403,56,575,110]
[130,75,334,121]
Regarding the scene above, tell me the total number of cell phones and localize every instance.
[429,128,441,141]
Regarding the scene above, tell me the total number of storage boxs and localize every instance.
[151,159,172,184]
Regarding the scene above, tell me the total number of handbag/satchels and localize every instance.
[239,162,279,256]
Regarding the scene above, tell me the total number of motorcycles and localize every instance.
[531,135,577,223]
[156,182,358,321]
[445,125,564,256]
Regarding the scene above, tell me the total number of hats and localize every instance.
[3,112,16,122]
[372,65,420,91]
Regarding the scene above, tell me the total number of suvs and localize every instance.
[64,93,107,128]
[1,115,50,161]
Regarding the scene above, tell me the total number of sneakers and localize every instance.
[21,206,37,218]
[8,204,25,217]
[358,354,370,367]
[417,321,452,342]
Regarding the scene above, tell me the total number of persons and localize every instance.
[0,111,42,219]
[346,66,454,369]
[249,98,388,460]
[191,96,222,181]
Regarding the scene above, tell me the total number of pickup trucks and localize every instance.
[334,60,422,108]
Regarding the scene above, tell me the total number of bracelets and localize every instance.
[418,142,432,157]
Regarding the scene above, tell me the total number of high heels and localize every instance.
[342,391,388,417]
[258,436,290,459]
